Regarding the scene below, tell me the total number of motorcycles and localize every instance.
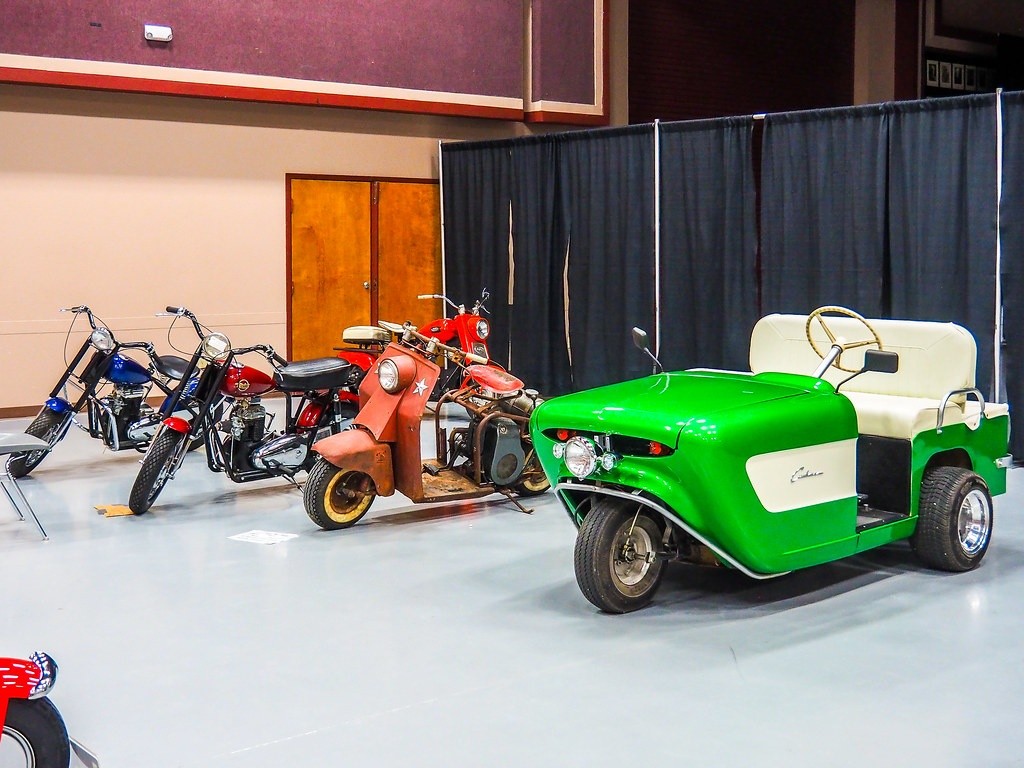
[5,304,223,479]
[128,305,361,517]
[0,652,100,768]
[336,287,507,403]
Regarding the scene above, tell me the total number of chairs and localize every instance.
[0,432,50,541]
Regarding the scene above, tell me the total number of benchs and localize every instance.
[749,314,978,442]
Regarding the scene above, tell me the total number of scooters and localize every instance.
[302,320,552,531]
